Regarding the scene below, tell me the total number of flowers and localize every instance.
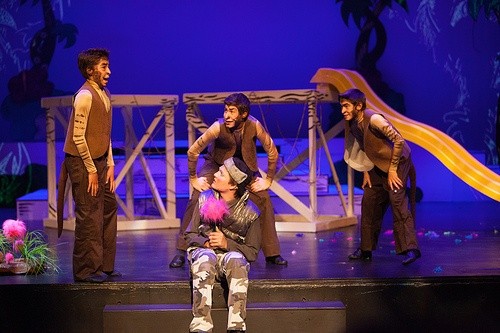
[0,219,62,276]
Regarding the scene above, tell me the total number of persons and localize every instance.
[184,156,262,333]
[337,88,424,266]
[168,93,289,267]
[56,48,123,283]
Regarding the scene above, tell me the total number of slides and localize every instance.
[309,67,500,203]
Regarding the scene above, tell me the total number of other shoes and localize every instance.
[105,271,123,278]
[89,272,108,283]
[189,316,213,333]
[227,313,246,331]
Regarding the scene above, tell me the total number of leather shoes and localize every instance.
[404,249,420,266]
[169,255,185,267]
[348,247,372,259]
[265,255,288,265]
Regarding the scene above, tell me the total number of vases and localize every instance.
[9,258,37,274]
